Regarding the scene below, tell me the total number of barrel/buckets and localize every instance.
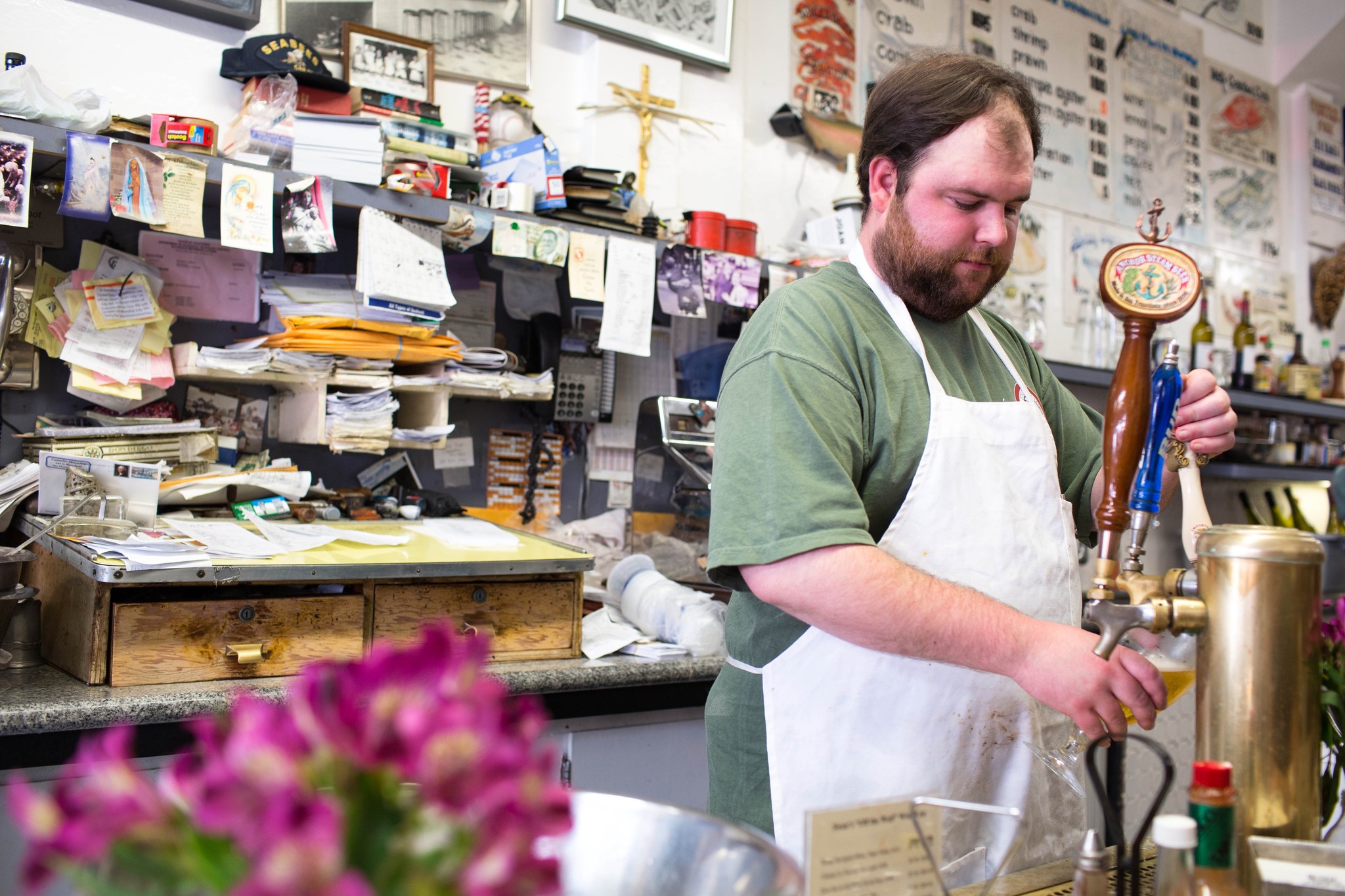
[725,219,758,257]
[683,210,726,250]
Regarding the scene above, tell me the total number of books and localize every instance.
[547,166,644,234]
[226,78,479,170]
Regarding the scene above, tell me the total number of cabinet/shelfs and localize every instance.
[0,117,1345,815]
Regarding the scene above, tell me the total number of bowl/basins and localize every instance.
[1314,535,1345,593]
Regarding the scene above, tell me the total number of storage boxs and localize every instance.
[293,116,386,185]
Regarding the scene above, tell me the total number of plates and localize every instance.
[1321,397,1345,408]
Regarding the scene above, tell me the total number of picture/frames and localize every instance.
[557,0,735,71]
[278,0,531,92]
[338,21,435,105]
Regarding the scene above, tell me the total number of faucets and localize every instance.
[1079,195,1202,661]
[1115,337,1186,608]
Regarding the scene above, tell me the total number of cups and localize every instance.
[1267,418,1342,466]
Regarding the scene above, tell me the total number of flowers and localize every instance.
[2,617,573,896]
[1316,590,1344,819]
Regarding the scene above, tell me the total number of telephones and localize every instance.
[534,309,603,422]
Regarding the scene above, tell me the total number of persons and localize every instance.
[701,46,1237,888]
[114,466,128,478]
[352,42,425,85]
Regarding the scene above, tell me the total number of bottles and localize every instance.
[1074,828,1111,896]
[1236,486,1345,535]
[1232,290,1345,401]
[1190,286,1215,376]
[1150,814,1200,896]
[1186,760,1248,896]
